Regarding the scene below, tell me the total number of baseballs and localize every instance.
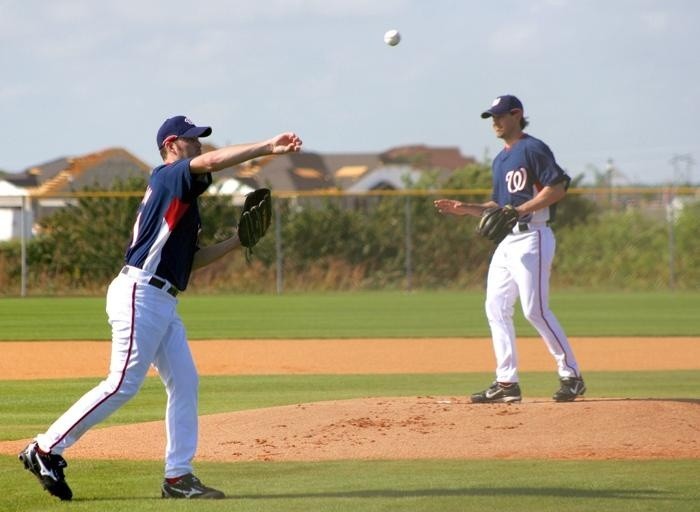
[386,29,401,45]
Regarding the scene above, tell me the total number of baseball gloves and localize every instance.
[238,188,271,247]
[476,204,518,243]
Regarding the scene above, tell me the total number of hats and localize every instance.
[157,115,212,150]
[481,95,523,119]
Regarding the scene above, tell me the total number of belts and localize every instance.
[121,267,179,298]
[508,220,552,231]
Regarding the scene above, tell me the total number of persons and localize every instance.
[18,114,303,500]
[431,94,589,403]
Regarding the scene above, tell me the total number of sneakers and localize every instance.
[469,384,520,403]
[554,376,588,401]
[159,472,225,501]
[17,441,73,502]
